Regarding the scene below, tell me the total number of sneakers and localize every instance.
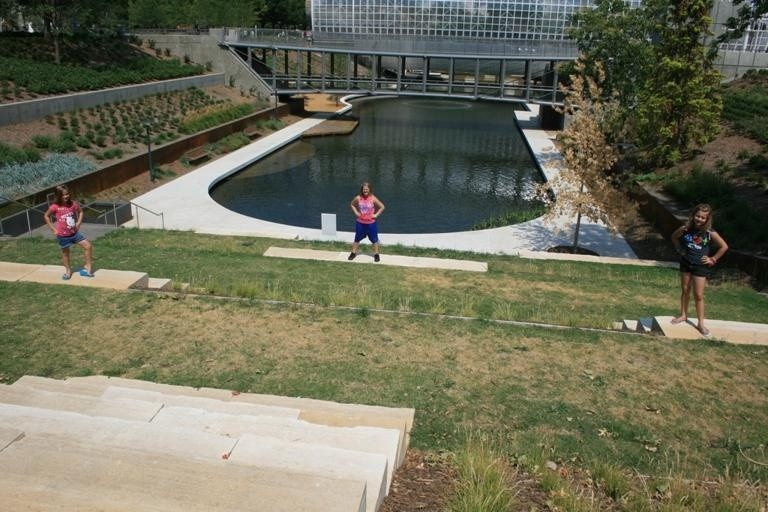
[374,254,379,262]
[349,252,355,260]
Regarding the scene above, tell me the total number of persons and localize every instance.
[44,184,94,280]
[193,23,313,46]
[347,182,385,261]
[671,203,728,336]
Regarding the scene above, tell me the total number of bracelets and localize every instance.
[375,214,379,218]
[711,257,717,263]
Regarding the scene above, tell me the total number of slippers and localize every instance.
[80,269,92,276]
[63,273,70,280]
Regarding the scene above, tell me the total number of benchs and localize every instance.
[242,125,258,139]
[185,145,211,166]
